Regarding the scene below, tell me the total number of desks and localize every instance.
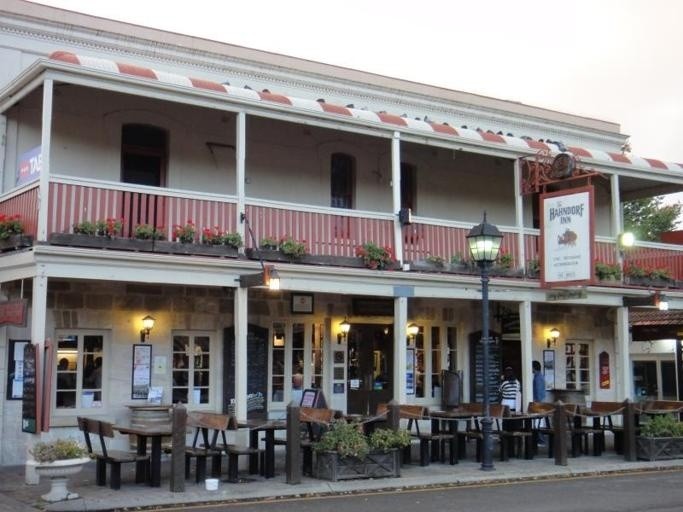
[119,414,182,486]
[338,408,376,434]
[436,404,488,461]
[506,403,537,462]
[572,401,605,460]
[239,411,287,482]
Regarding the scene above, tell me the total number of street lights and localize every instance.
[466,211,504,475]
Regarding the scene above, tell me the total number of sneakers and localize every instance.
[537,441,546,447]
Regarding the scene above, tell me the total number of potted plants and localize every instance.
[312,419,369,482]
[30,439,96,505]
[370,424,415,478]
[676,424,683,461]
[642,416,675,461]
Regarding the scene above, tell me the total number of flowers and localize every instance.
[277,232,292,253]
[357,243,377,260]
[170,220,183,246]
[295,235,309,255]
[498,249,512,271]
[643,261,658,276]
[624,260,642,278]
[380,244,397,263]
[592,257,614,281]
[184,220,195,249]
[201,224,212,248]
[153,222,169,243]
[0,213,22,232]
[262,234,275,252]
[97,214,120,237]
[212,221,227,247]
[658,262,675,277]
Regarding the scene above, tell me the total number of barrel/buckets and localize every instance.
[205,477,220,491]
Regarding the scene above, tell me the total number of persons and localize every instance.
[88,357,101,400]
[291,372,303,391]
[498,367,521,411]
[56,358,73,406]
[531,360,548,447]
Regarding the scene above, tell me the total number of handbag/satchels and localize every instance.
[515,391,522,412]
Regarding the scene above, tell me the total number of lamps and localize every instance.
[232,266,286,294]
[404,321,421,340]
[619,232,638,252]
[650,293,676,316]
[545,328,565,348]
[137,315,157,339]
[338,318,353,346]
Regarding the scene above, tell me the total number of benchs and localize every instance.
[74,417,147,494]
[528,399,571,459]
[368,403,435,470]
[557,402,584,454]
[206,407,258,486]
[590,401,622,451]
[281,408,330,474]
[462,401,507,464]
[168,406,223,486]
